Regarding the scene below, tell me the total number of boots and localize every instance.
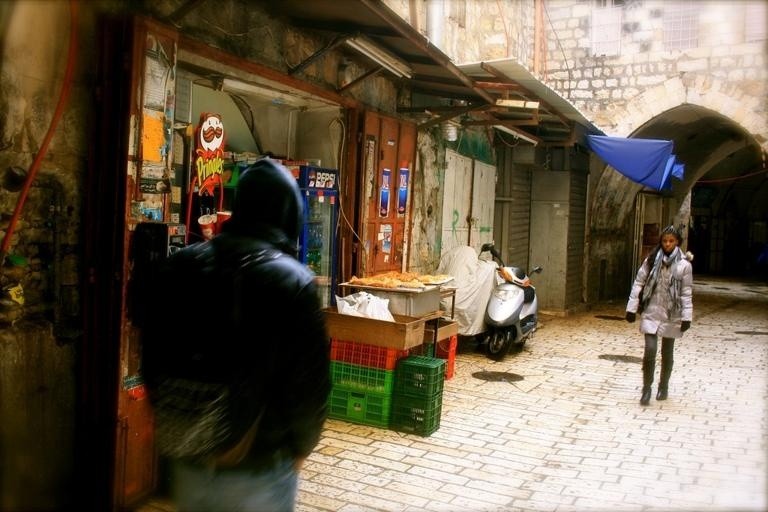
[656,361,674,400]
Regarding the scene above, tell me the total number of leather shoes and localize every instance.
[639,360,655,405]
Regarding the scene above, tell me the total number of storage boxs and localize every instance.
[317,304,458,437]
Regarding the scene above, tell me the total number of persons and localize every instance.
[626,227,694,405]
[140,158,332,512]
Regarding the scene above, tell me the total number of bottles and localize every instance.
[306,197,324,276]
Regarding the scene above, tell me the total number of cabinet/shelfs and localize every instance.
[293,164,338,321]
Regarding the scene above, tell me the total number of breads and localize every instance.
[349,270,450,288]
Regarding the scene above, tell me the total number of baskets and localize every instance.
[326,336,457,438]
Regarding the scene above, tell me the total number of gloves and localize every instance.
[679,321,693,333]
[627,311,635,323]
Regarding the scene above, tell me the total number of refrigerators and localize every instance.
[298,165,339,308]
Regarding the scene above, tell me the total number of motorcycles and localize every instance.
[480,243,543,360]
